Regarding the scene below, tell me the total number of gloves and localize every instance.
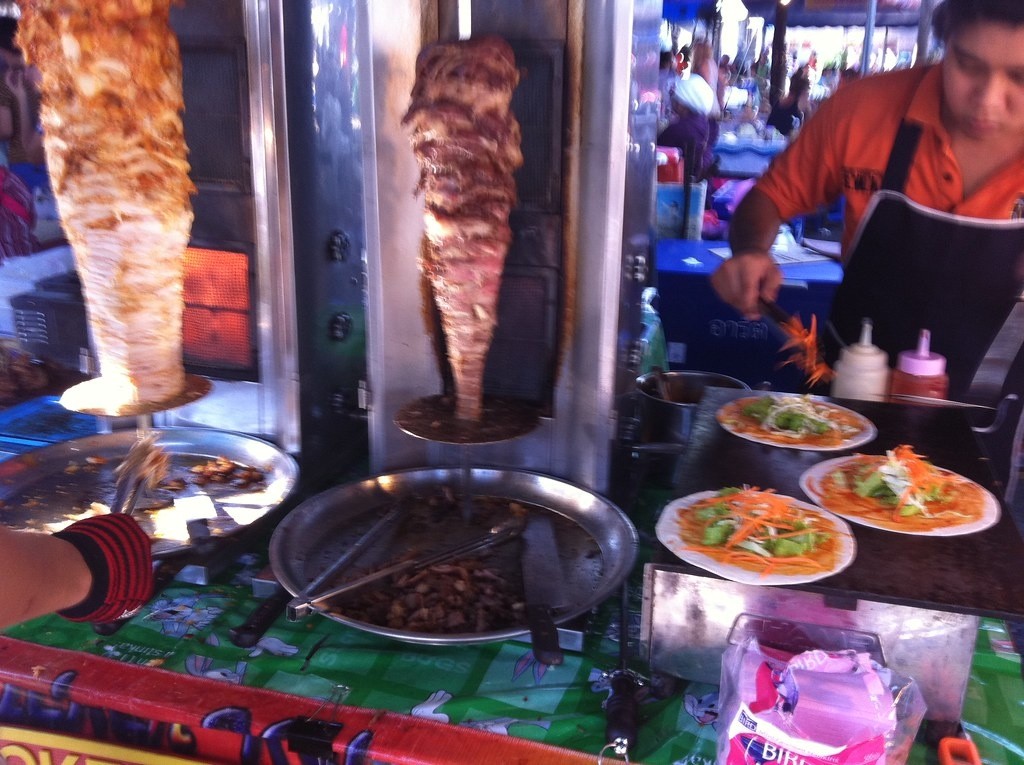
[53,515,154,623]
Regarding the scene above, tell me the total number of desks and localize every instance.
[652,238,843,392]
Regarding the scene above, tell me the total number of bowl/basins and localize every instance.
[635,370,752,443]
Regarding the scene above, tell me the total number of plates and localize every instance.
[716,396,876,450]
[798,455,1001,537]
[0,426,300,556]
[267,465,640,646]
[656,490,855,586]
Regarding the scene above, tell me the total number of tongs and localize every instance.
[111,431,169,515]
[289,490,526,616]
[759,295,833,382]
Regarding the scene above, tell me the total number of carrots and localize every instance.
[672,399,985,581]
[778,313,845,388]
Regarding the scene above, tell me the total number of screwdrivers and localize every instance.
[605,581,635,757]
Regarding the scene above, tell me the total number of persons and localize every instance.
[0,513,155,629]
[0,14,47,265]
[705,0,1024,385]
[659,41,861,178]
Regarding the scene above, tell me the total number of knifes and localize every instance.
[175,484,218,553]
[521,516,569,666]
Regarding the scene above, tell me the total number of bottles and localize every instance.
[830,323,891,402]
[888,329,949,405]
